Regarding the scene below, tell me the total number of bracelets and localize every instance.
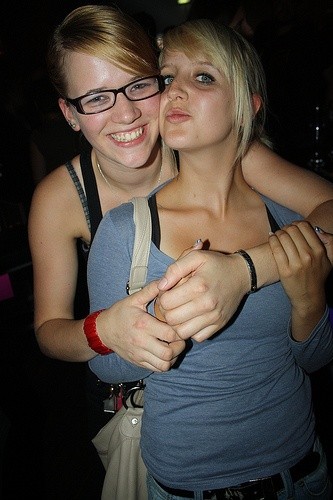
[233,248,257,294]
[83,309,115,355]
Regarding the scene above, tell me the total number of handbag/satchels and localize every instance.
[90,385,144,500]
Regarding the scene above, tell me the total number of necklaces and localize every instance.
[96,148,164,200]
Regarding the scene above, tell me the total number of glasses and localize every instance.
[61,74,166,116]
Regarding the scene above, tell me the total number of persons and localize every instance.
[87,19,333,500]
[27,5,333,500]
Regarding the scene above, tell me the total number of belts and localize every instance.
[151,439,323,499]
